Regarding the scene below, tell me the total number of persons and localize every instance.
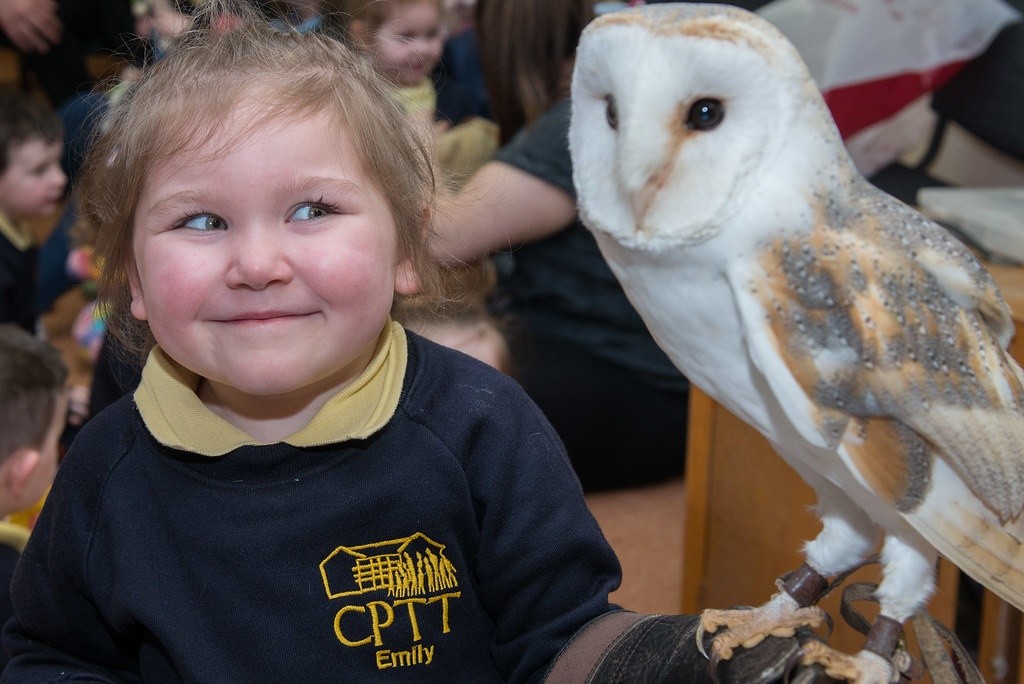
[0,1,1024,626]
[0,22,910,684]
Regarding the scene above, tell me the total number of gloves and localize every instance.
[583,615,845,684]
